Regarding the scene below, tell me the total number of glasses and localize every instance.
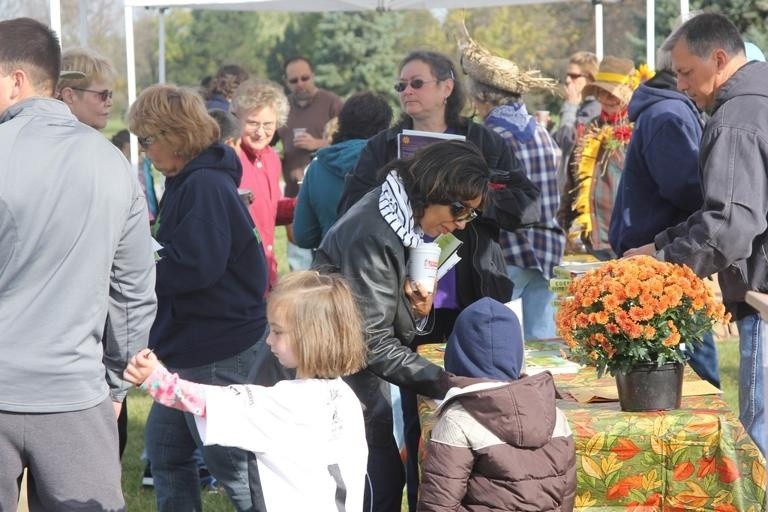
[59,86,114,100]
[566,73,588,79]
[450,200,479,223]
[246,122,275,130]
[394,80,435,93]
[137,133,163,147]
[288,75,311,84]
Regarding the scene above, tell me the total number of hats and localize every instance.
[582,55,655,105]
[463,42,565,96]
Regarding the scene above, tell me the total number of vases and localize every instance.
[612,360,684,411]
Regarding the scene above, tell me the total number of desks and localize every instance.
[414,341,767,511]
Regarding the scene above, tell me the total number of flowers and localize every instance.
[632,63,655,90]
[551,254,733,381]
[602,123,632,150]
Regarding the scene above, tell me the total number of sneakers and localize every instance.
[143,461,154,488]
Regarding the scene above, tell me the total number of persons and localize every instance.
[620,12,768,462]
[106,44,767,511]
[0,15,161,512]
[53,48,129,512]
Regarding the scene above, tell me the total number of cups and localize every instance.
[292,127,306,139]
[409,242,441,295]
[534,110,550,129]
